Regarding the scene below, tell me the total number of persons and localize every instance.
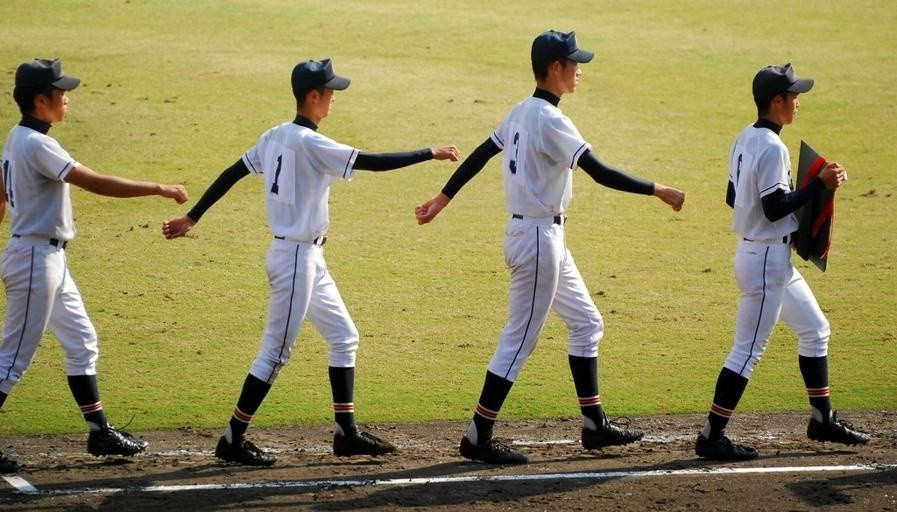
[1,59,191,474]
[415,30,685,463]
[161,59,463,469]
[696,63,872,458]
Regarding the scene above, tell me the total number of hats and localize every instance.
[532,30,594,63]
[292,58,350,90]
[15,59,80,89]
[753,63,814,92]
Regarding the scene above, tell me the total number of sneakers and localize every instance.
[582,424,645,449]
[695,436,759,460]
[334,431,398,457]
[460,437,527,464]
[215,436,278,466]
[807,411,870,444]
[88,424,150,457]
[0,451,20,474]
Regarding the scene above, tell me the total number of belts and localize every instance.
[512,214,567,224]
[744,233,793,244]
[274,235,326,246]
[12,234,68,249]
